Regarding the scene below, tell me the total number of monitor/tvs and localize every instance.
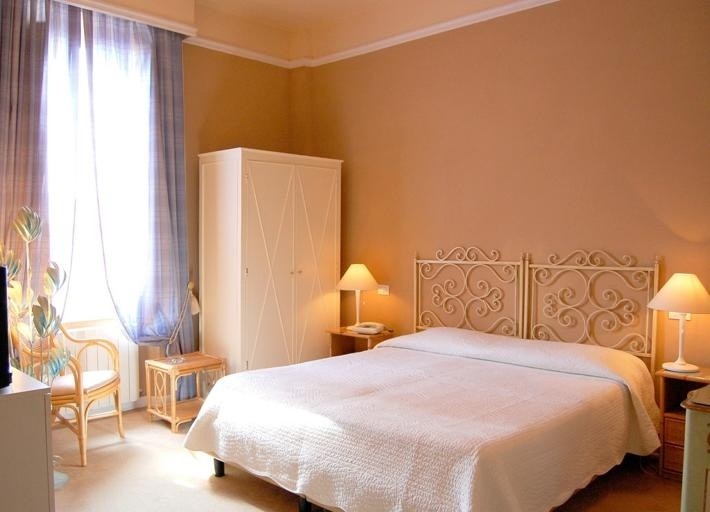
[0,266,11,390]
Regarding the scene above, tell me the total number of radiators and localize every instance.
[41,327,141,420]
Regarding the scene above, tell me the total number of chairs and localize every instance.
[11,308,124,467]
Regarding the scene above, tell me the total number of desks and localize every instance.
[679,386,710,512]
[145,351,226,433]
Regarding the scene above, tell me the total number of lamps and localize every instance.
[644,272,710,373]
[335,263,378,330]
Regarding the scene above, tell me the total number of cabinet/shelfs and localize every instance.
[0,365,55,512]
[198,145,343,373]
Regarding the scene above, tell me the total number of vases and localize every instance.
[14,329,53,377]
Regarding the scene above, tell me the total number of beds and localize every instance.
[184,245,663,512]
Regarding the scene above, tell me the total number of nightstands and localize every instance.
[655,363,710,482]
[326,325,394,357]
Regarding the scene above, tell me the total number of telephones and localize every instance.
[347,322,384,334]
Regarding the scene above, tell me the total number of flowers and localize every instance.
[0,205,71,388]
